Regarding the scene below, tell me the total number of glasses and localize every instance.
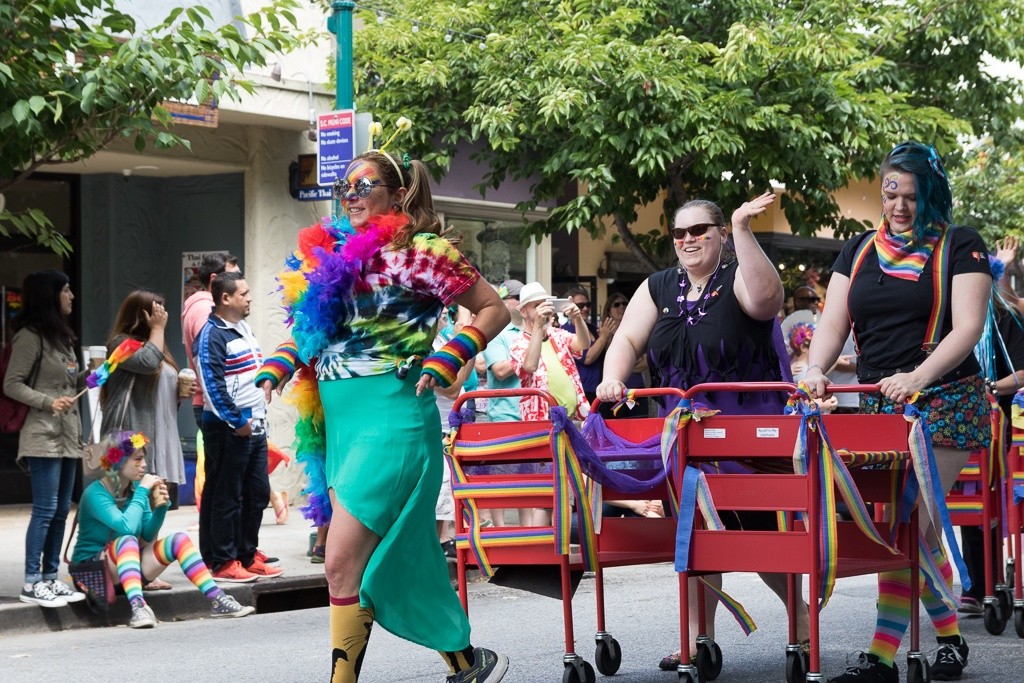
[670,223,724,239]
[614,301,628,307]
[576,302,591,309]
[333,175,399,200]
[504,295,519,300]
[799,296,821,304]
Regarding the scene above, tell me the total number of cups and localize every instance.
[178,366,198,398]
[88,344,107,375]
[148,478,169,508]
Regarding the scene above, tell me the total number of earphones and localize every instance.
[720,235,724,244]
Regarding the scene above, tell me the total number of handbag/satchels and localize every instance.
[1,326,44,434]
[82,444,106,491]
[68,559,118,614]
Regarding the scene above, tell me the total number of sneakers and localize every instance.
[212,560,260,582]
[256,549,281,567]
[445,647,510,683]
[930,634,969,681]
[44,579,86,602]
[19,581,68,608]
[247,556,285,578]
[129,596,157,628]
[209,589,255,618]
[826,653,899,683]
[311,544,325,563]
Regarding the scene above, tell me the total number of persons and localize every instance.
[195,271,280,582]
[254,147,513,683]
[100,292,198,500]
[67,429,254,626]
[397,235,1024,615]
[181,252,282,576]
[307,511,328,564]
[797,139,997,680]
[594,188,814,673]
[2,268,99,609]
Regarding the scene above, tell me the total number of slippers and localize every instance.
[275,491,289,525]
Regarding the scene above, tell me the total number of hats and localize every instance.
[516,282,557,312]
[498,279,524,300]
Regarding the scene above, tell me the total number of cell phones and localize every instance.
[547,299,571,312]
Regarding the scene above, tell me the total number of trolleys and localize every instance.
[994,382,1024,639]
[441,384,729,683]
[895,384,1005,630]
[669,378,937,681]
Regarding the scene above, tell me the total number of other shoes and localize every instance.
[659,651,697,671]
[144,581,172,590]
[957,593,984,614]
